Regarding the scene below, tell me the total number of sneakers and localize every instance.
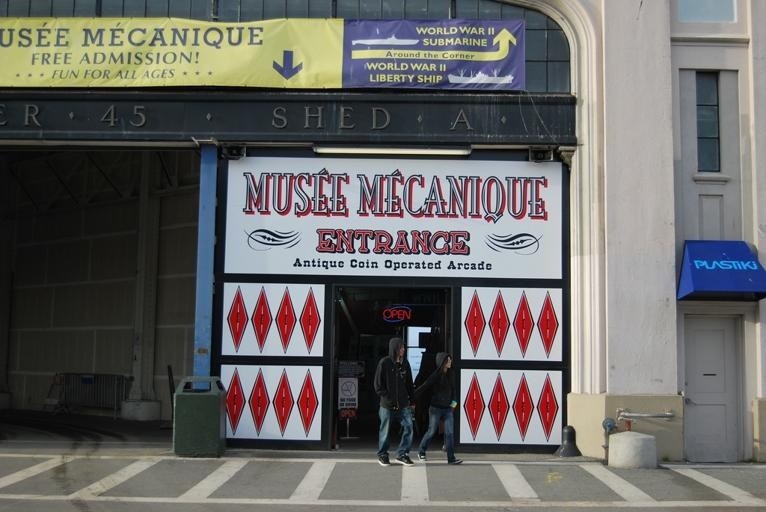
[448,460,463,464]
[396,455,414,466]
[378,455,391,467]
[418,453,427,461]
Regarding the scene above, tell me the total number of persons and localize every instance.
[415,353,463,464]
[372,337,416,467]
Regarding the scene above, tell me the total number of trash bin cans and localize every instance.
[173,376,227,458]
